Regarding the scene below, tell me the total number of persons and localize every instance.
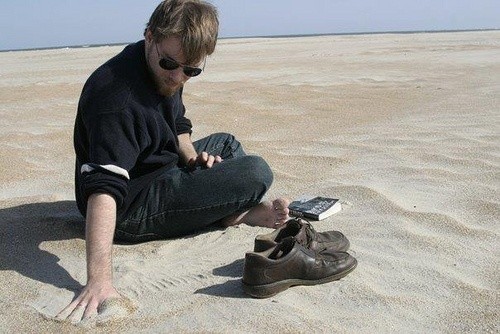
[56,0,289,321]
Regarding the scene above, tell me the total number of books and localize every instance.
[288,193,342,221]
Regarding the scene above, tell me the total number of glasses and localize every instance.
[156,41,207,77]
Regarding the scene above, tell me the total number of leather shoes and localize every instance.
[254,216,351,251]
[239,236,358,299]
